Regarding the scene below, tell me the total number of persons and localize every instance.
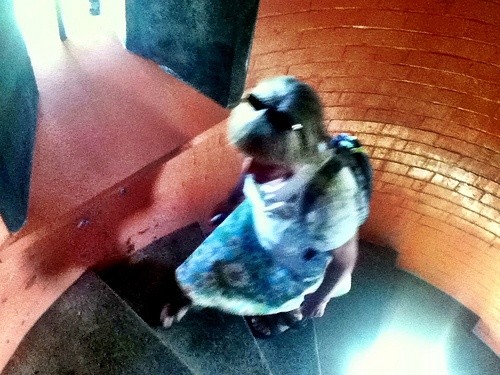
[160,75,370,340]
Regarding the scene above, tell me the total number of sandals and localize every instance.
[158,300,196,331]
[249,312,309,339]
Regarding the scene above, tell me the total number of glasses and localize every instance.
[242,91,305,134]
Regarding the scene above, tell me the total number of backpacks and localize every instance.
[298,131,373,261]
